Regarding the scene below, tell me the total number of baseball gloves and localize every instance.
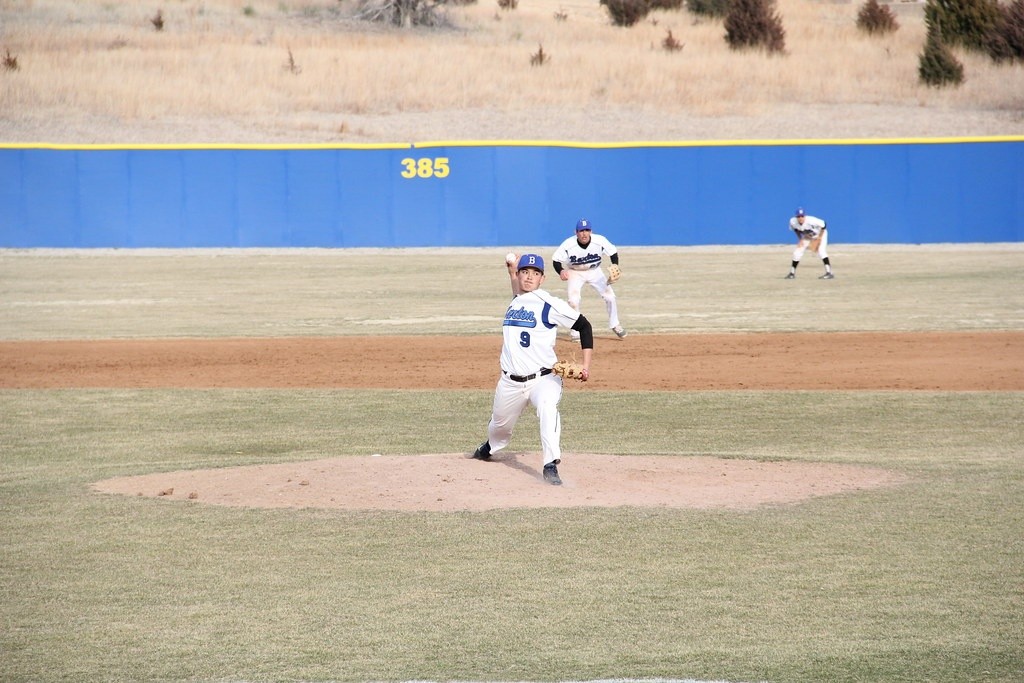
[552,361,584,380]
[506,253,516,263]
[607,265,621,286]
[807,238,821,252]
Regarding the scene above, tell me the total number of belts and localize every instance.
[501,367,552,382]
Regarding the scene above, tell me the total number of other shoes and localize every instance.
[784,272,795,280]
[819,272,835,279]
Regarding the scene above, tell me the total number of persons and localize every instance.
[552,220,629,340]
[472,254,594,486]
[781,206,832,281]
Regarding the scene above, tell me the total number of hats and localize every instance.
[575,218,592,231]
[516,253,545,276]
[794,206,805,217]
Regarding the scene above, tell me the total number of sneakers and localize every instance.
[571,336,581,343]
[612,324,628,339]
[542,464,562,486]
[472,440,492,462]
